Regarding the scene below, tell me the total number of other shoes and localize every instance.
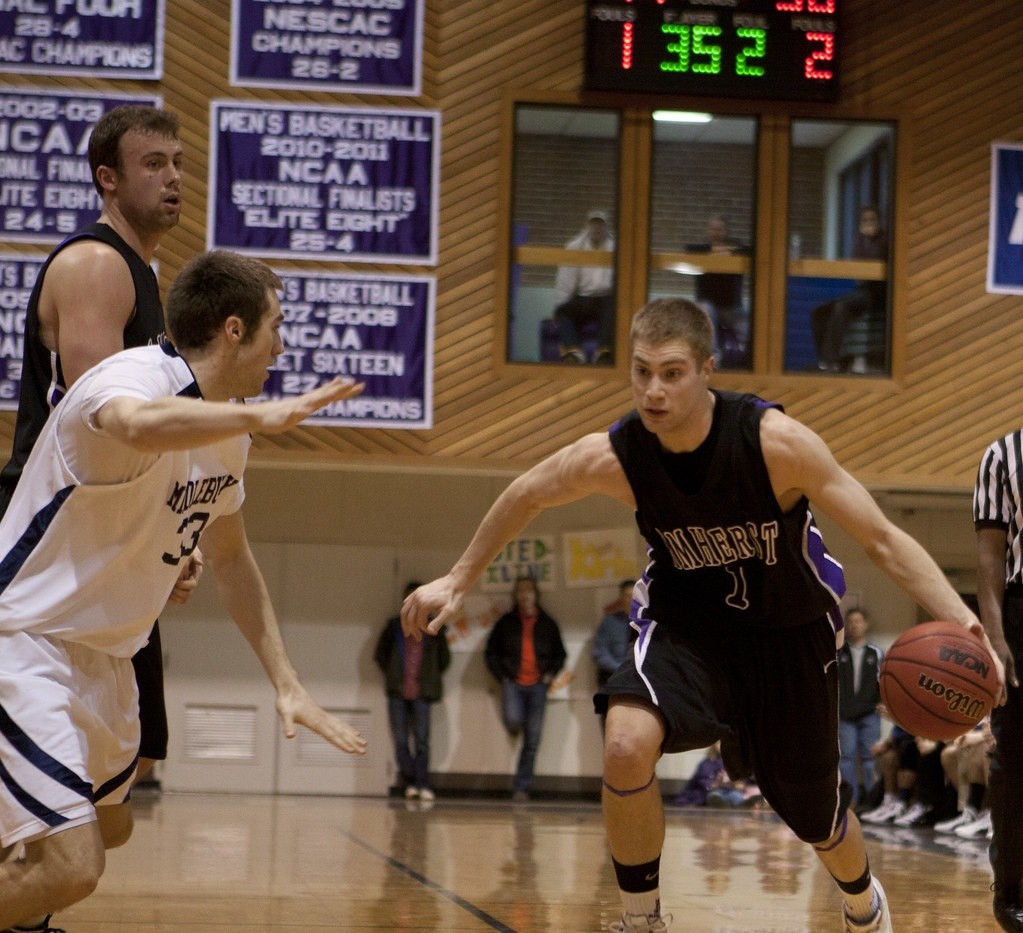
[513,789,528,807]
[405,789,434,800]
[910,808,961,829]
[560,347,611,364]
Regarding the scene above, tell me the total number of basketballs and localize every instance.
[880,621,998,741]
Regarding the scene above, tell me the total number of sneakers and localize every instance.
[860,792,931,827]
[609,913,673,933]
[933,808,994,840]
[0,912,66,933]
[841,874,893,933]
[990,875,1023,933]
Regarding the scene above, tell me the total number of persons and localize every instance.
[682,218,752,371]
[553,212,618,365]
[1,106,206,787]
[972,428,1023,933]
[680,740,770,811]
[401,297,1007,865]
[589,580,639,732]
[0,251,368,933]
[484,575,568,802]
[837,607,994,842]
[809,209,892,372]
[374,580,452,800]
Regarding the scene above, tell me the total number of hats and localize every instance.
[587,211,609,226]
